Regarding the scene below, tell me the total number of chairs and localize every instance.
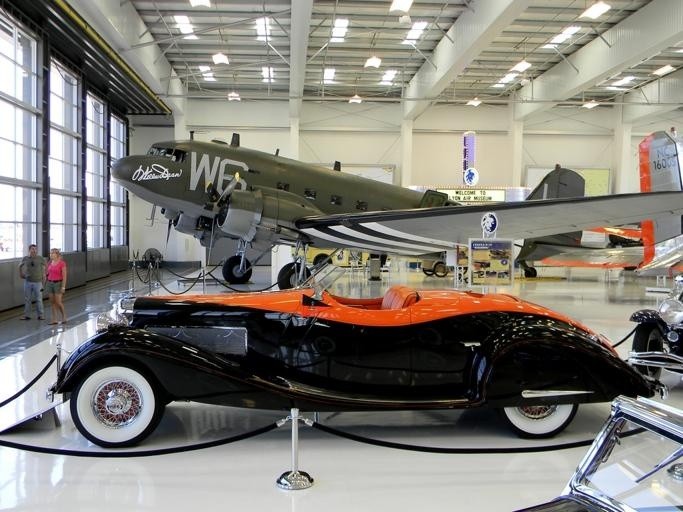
[380,286,417,310]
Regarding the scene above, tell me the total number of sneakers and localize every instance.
[37,316,46,320]
[19,316,31,320]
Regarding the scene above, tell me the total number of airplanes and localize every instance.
[110,129,683,290]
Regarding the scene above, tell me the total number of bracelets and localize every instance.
[61,287,65,290]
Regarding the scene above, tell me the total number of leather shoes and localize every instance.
[61,319,66,324]
[48,321,58,325]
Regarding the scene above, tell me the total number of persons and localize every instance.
[16,244,48,320]
[44,247,68,326]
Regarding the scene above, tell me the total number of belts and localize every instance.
[48,280,62,283]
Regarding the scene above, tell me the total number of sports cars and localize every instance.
[48,263,667,449]
